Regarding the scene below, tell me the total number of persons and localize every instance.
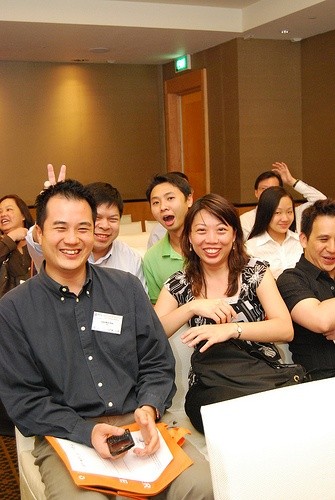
[276,197,335,381]
[0,193,38,301]
[0,179,214,500]
[241,185,304,281]
[155,193,311,436]
[237,161,326,243]
[143,172,194,310]
[25,164,151,302]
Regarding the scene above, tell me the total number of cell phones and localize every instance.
[107,429,135,455]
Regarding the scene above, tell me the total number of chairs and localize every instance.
[14,214,293,500]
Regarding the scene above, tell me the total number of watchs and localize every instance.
[232,321,242,341]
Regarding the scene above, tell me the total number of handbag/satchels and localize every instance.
[191,338,305,393]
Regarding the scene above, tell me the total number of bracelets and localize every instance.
[138,403,160,423]
[292,178,300,189]
